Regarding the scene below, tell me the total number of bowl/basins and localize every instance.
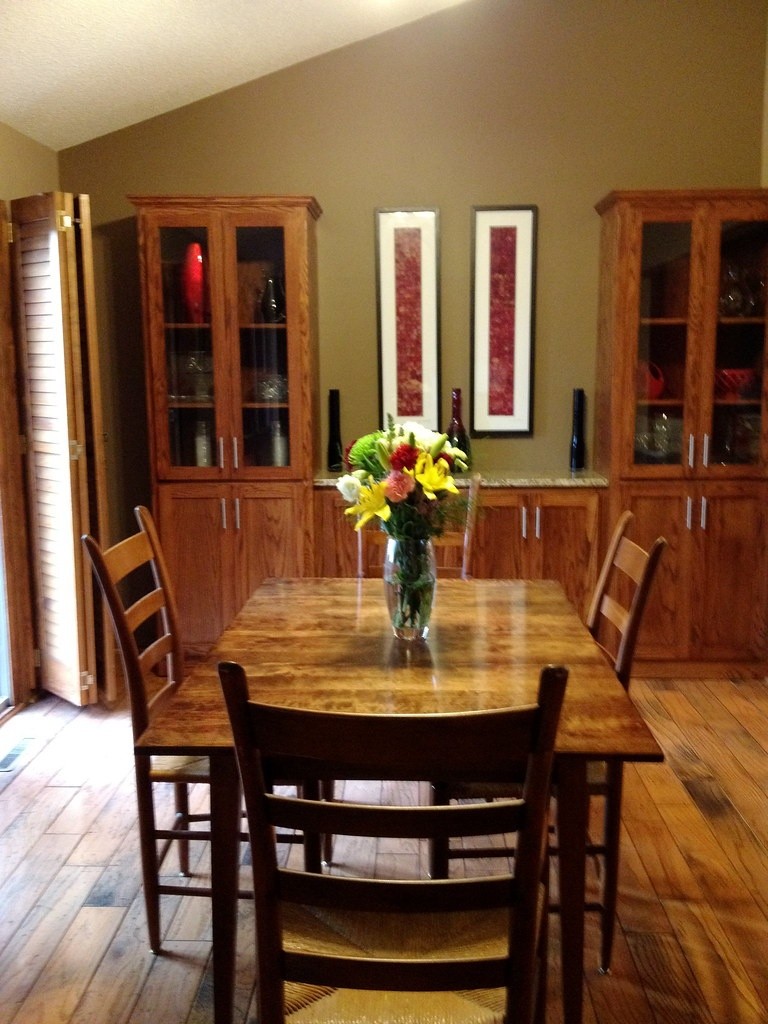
[714,369,759,400]
[725,414,761,459]
[635,414,685,456]
[253,374,287,402]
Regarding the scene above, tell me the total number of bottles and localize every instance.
[185,351,213,402]
[195,421,213,467]
[328,389,343,472]
[570,388,586,471]
[446,388,473,473]
[184,242,207,323]
[260,268,285,323]
[271,421,289,466]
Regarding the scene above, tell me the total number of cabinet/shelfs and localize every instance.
[593,188,768,481]
[125,191,323,481]
[446,489,610,626]
[610,482,768,661]
[313,489,444,578]
[152,482,313,677]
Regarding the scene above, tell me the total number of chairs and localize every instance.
[216,660,569,1024]
[79,505,336,957]
[429,510,666,976]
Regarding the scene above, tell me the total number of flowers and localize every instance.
[335,413,478,630]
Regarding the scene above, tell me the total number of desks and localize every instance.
[131,575,665,1024]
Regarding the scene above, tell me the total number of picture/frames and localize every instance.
[469,205,539,441]
[372,207,442,435]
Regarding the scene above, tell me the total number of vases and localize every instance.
[445,387,473,474]
[253,368,288,403]
[178,351,212,397]
[189,421,216,467]
[569,388,585,473]
[713,369,760,400]
[259,255,285,323]
[329,389,343,472]
[383,537,436,641]
[261,420,288,467]
[178,241,208,324]
